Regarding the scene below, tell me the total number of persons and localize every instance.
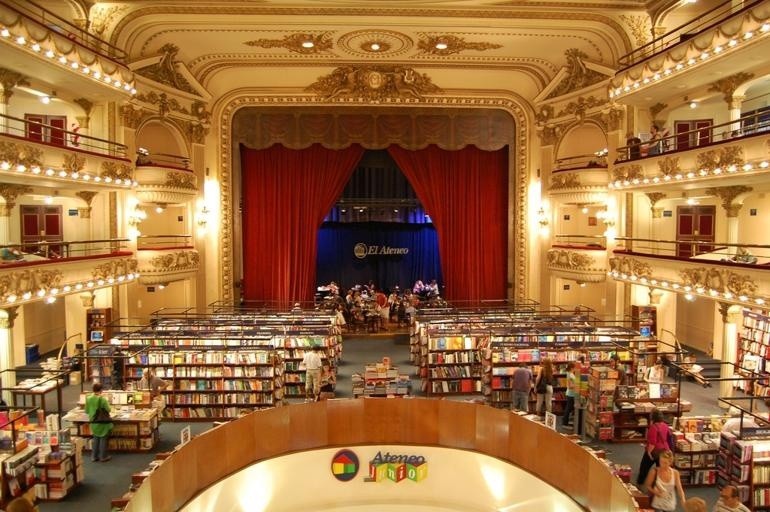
[33,241,65,260]
[731,130,742,138]
[624,131,642,160]
[0,242,26,262]
[639,125,664,156]
[720,131,730,140]
[313,279,439,330]
[735,245,756,263]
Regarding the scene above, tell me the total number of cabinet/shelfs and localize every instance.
[407,303,770,512]
[0,301,343,512]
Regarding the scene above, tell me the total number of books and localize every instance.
[1,314,344,512]
[349,357,412,398]
[410,311,770,511]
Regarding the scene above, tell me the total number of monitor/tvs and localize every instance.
[640,326,650,337]
[91,331,103,341]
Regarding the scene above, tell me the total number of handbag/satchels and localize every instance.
[92,396,113,424]
[534,368,547,394]
[668,430,676,454]
[646,467,658,498]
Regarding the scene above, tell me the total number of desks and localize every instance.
[317,275,446,333]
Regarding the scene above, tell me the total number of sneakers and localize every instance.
[91,455,112,461]
